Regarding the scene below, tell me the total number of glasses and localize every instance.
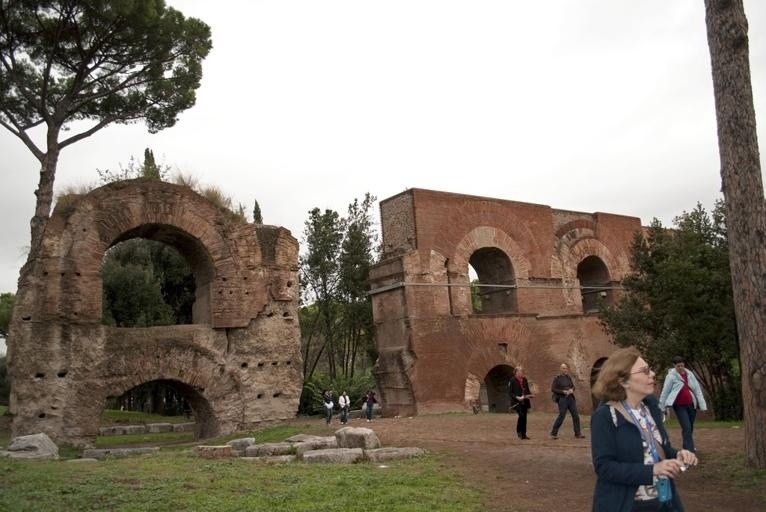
[623,366,652,375]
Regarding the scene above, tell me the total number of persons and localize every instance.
[322,388,334,426]
[338,389,351,425]
[362,389,376,423]
[659,354,707,452]
[589,344,698,511]
[549,363,585,439]
[508,366,534,440]
